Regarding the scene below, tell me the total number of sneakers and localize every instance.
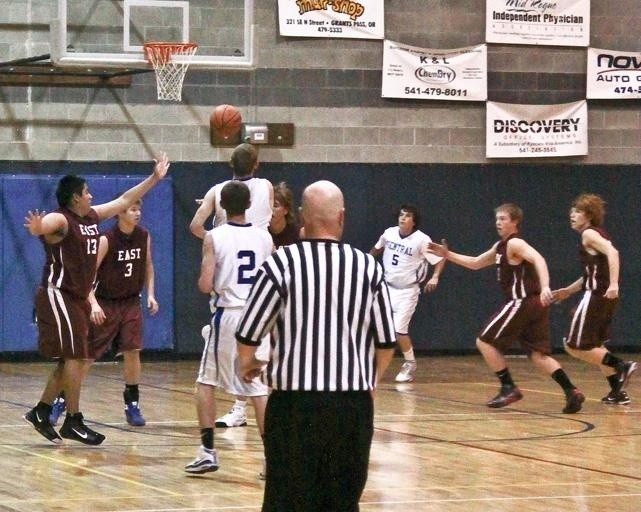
[49,397,65,426]
[22,408,63,445]
[615,360,637,393]
[395,359,417,382]
[563,388,585,413]
[602,389,631,404]
[215,405,247,427]
[184,445,218,473]
[125,401,145,426]
[59,417,105,446]
[486,385,522,408]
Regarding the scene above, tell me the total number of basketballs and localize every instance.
[212,105,242,140]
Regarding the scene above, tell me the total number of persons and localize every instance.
[426,203,586,414]
[212,181,300,428]
[547,192,637,404]
[49,191,159,427]
[23,149,172,448]
[188,143,275,239]
[185,180,276,480]
[367,203,448,383]
[234,179,397,511]
